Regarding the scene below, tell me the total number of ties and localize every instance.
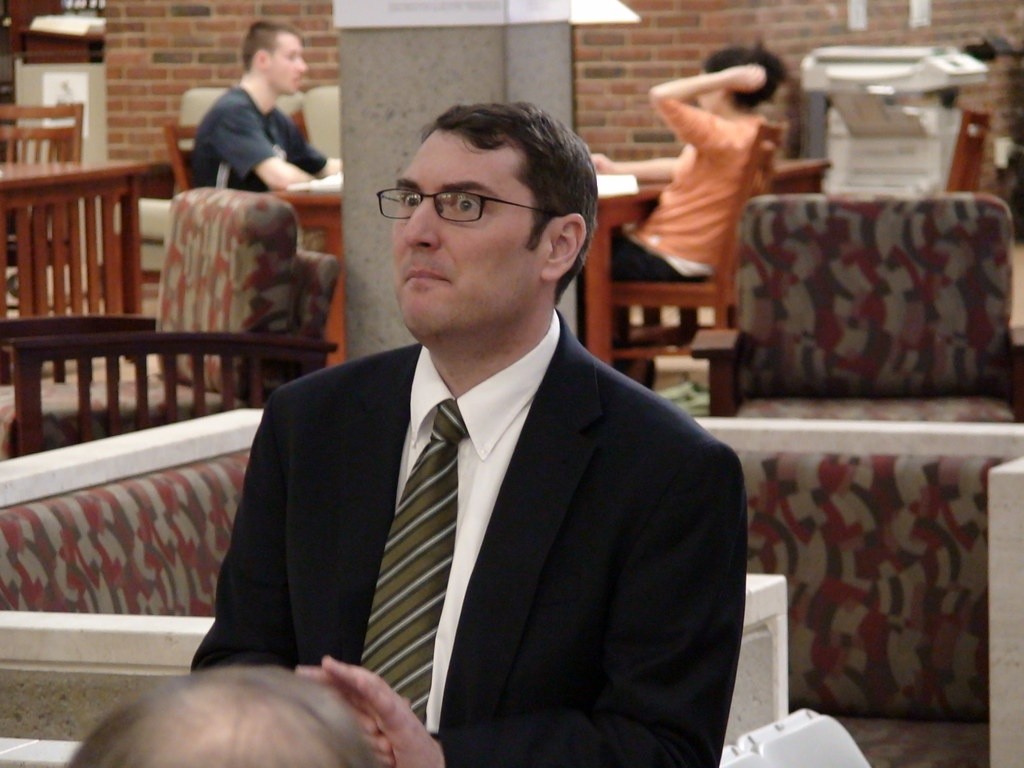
[359,399,470,725]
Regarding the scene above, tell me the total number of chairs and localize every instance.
[0,101,1024,768]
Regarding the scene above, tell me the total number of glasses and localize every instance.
[375,188,552,222]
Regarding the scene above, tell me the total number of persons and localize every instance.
[573,45,788,391]
[188,16,344,193]
[70,663,381,768]
[187,100,749,768]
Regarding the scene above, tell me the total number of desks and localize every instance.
[258,179,344,367]
[568,153,829,371]
[2,158,146,315]
